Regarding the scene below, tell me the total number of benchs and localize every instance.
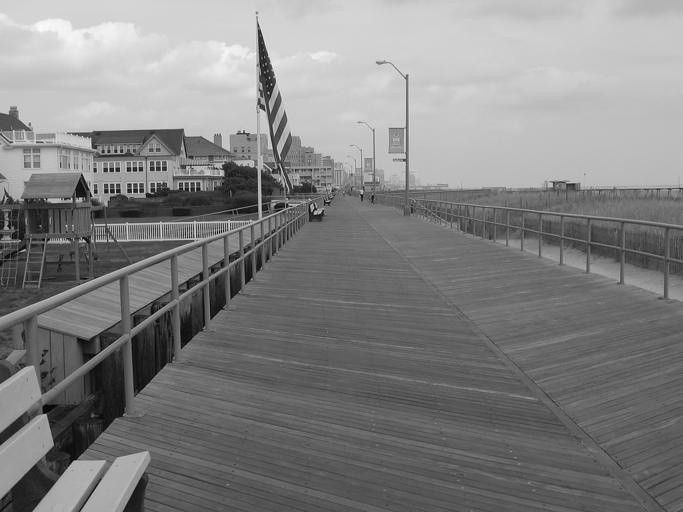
[0,366,151,512]
[309,198,331,222]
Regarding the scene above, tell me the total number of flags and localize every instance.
[256,22,294,195]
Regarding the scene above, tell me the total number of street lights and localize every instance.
[357,121,375,189]
[345,145,362,189]
[376,61,410,216]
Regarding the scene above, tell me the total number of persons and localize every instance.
[369,188,375,204]
[359,188,363,202]
